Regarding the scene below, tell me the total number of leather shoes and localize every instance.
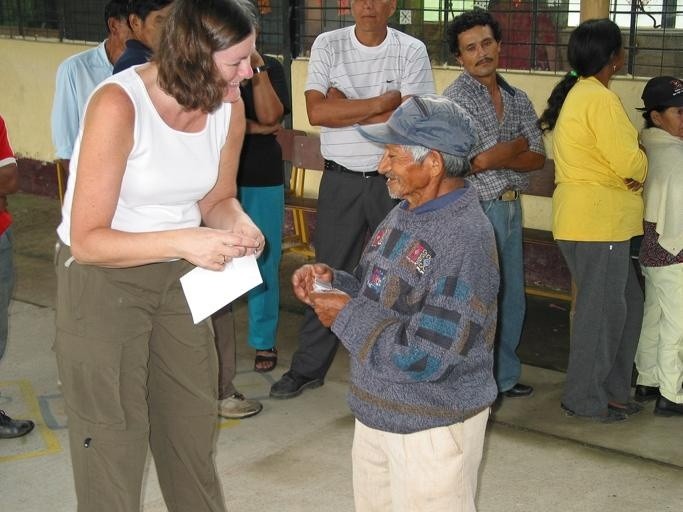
[654,395,683,417]
[503,384,533,398]
[562,399,644,423]
[270,372,324,400]
[635,386,659,402]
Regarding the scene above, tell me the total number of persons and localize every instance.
[536,19,648,424]
[441,10,545,396]
[633,76,683,417]
[57,0,266,512]
[52,0,289,419]
[269,1,437,399]
[0,117,35,439]
[289,97,507,512]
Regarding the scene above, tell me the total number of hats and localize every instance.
[357,96,479,157]
[642,77,683,109]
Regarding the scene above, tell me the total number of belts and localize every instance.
[495,188,520,200]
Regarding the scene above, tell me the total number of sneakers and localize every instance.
[218,392,262,418]
[0,410,33,437]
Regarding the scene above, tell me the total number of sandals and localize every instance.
[254,348,278,371]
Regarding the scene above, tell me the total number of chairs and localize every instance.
[495,156,578,338]
[270,130,305,236]
[284,136,321,258]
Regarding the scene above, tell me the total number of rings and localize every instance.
[217,254,225,267]
